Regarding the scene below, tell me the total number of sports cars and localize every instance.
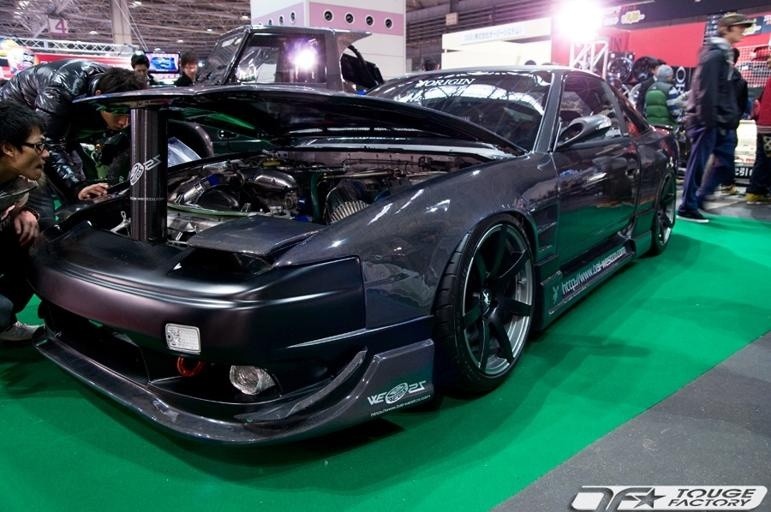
[20,62,683,448]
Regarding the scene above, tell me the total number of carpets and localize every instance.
[0,205,771,512]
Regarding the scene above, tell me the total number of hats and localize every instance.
[718,11,754,28]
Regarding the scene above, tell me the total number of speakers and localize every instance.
[673,66,694,93]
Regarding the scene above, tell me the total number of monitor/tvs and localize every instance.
[144,51,182,74]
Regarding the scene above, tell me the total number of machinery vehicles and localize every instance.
[49,21,348,219]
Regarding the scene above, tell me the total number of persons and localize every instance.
[131,54,152,85]
[174,51,204,87]
[2,57,146,231]
[423,57,440,71]
[628,10,770,225]
[2,95,46,351]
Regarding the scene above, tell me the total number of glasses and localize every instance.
[23,141,45,155]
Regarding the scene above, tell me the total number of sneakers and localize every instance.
[721,186,738,195]
[678,205,709,223]
[696,193,716,201]
[746,193,771,204]
[1,320,46,341]
